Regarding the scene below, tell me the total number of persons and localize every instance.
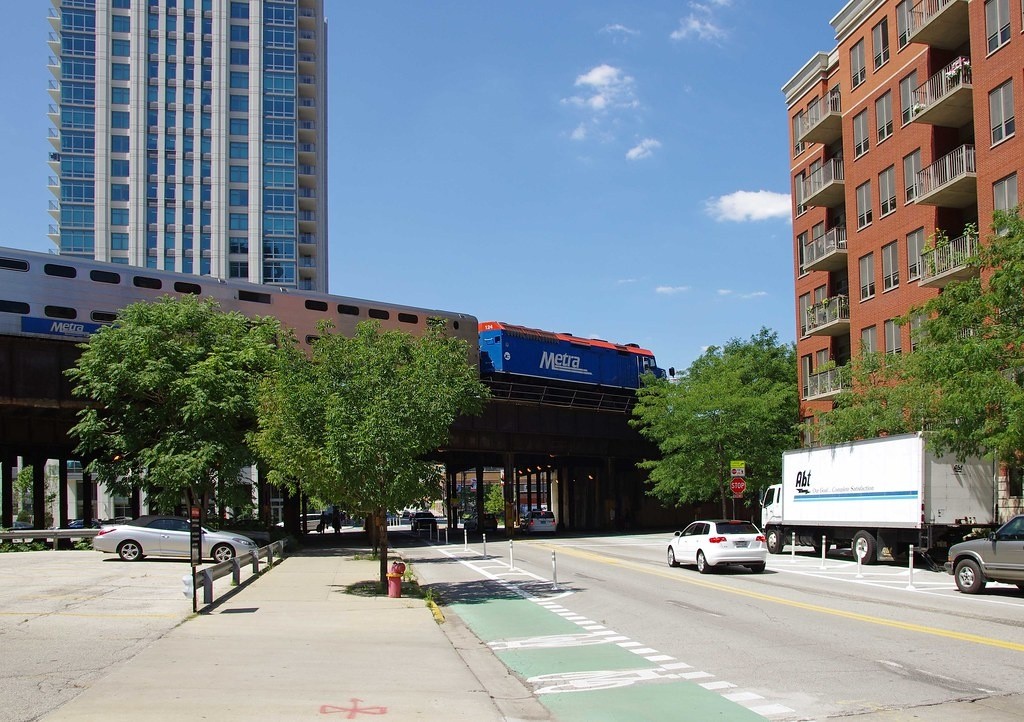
[314,508,355,533]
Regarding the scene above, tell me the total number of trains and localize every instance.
[0,247,679,394]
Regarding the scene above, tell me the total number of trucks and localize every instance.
[759,430,999,565]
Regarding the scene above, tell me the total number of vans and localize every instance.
[300,514,325,532]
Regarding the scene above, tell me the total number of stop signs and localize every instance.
[729,478,747,495]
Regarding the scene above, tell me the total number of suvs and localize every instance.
[411,512,437,533]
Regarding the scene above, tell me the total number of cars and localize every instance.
[520,511,557,534]
[385,510,416,521]
[91,516,259,564]
[943,514,1024,594]
[666,518,768,575]
[217,520,269,531]
[463,513,497,531]
[51,517,133,529]
[12,522,34,531]
